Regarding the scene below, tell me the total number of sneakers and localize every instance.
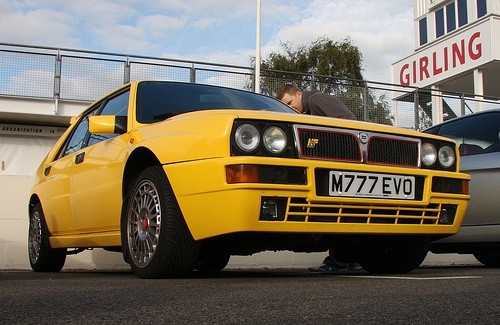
[308,257,360,273]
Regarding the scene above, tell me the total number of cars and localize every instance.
[26,78,472,279]
[420,107,500,269]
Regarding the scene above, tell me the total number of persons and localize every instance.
[276,84,361,273]
